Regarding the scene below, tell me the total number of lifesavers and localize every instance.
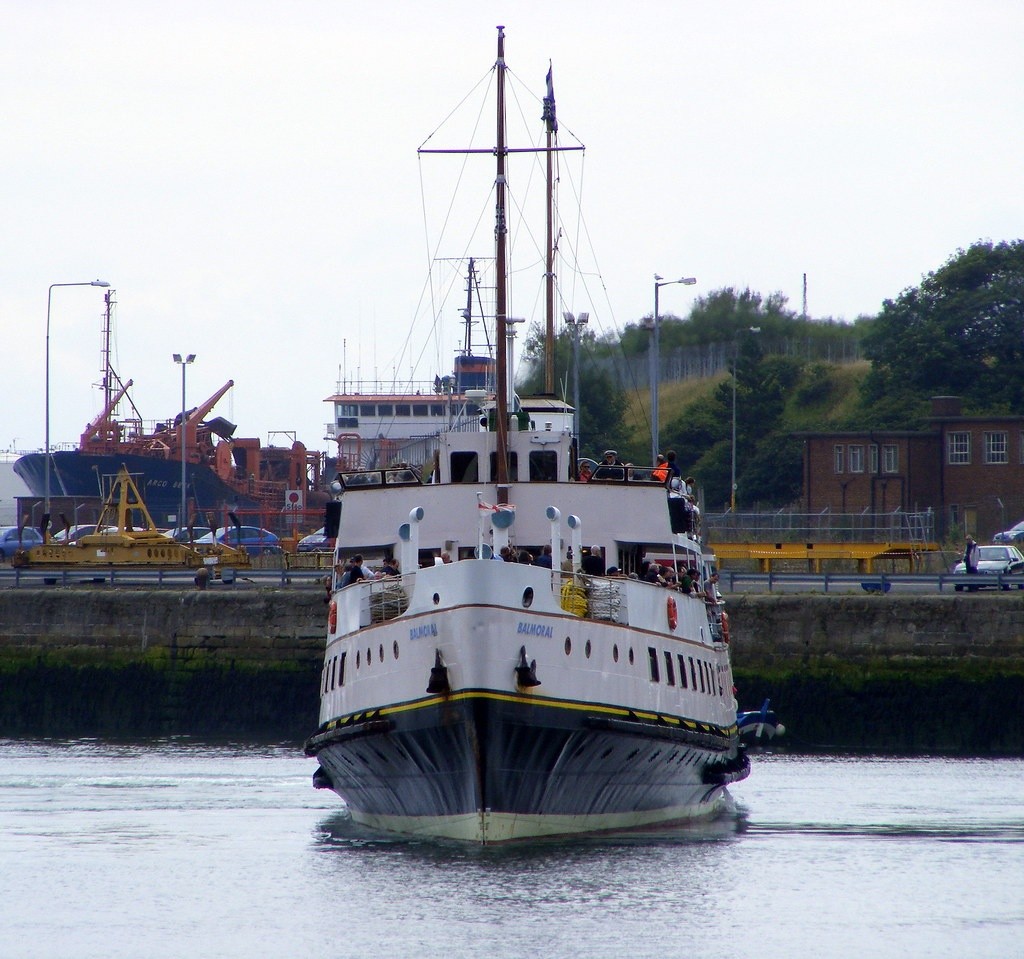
[722,612,729,643]
[668,596,677,630]
[329,601,337,634]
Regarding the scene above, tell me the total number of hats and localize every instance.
[604,450,618,456]
[607,567,622,574]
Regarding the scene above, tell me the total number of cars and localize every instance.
[992,521,1023,544]
[0,526,325,562]
[954,546,1024,591]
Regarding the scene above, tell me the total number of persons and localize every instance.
[347,463,413,483]
[562,545,605,577]
[599,450,623,479]
[334,555,374,591]
[625,463,634,480]
[704,573,719,605]
[195,564,207,586]
[689,530,703,544]
[642,561,705,598]
[607,566,638,579]
[651,451,700,516]
[578,461,593,480]
[442,552,453,564]
[492,543,552,568]
[374,558,399,580]
[963,535,980,592]
[706,608,712,617]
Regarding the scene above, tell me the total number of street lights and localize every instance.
[733,325,760,516]
[173,354,196,527]
[653,273,697,471]
[565,313,589,459]
[46,280,112,512]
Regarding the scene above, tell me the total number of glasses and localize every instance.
[349,560,354,564]
[607,455,615,458]
[581,466,590,469]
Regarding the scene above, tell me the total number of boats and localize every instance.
[13,287,348,538]
[303,25,751,846]
